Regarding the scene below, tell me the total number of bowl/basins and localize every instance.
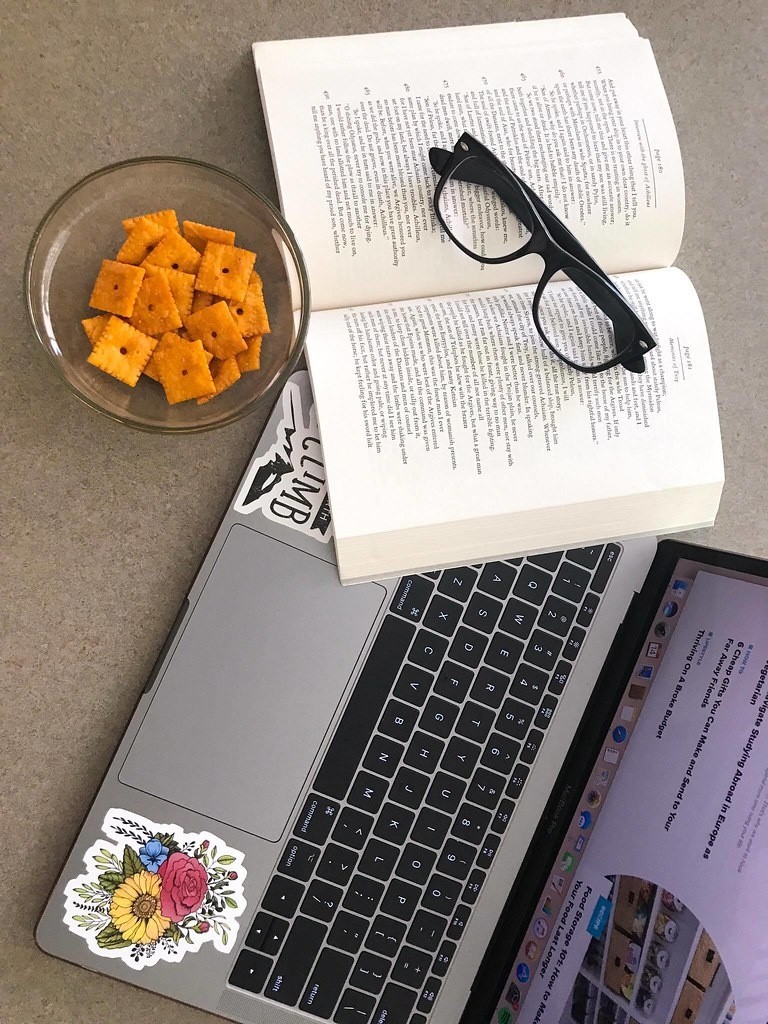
[22,157,311,436]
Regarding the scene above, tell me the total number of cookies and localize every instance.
[82,210,269,407]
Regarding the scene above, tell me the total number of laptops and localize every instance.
[32,370,768,1024]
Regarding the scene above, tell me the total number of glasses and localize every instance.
[428,132,657,375]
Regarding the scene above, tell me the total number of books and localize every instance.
[251,11,725,588]
